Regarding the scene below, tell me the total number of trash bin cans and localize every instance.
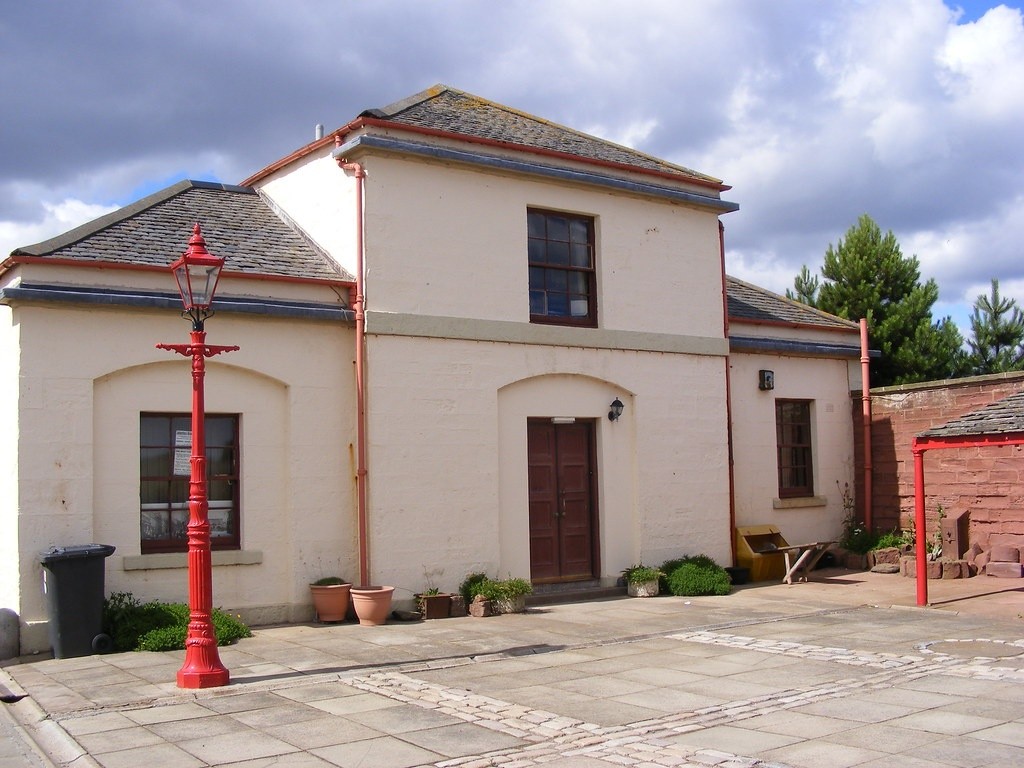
[39,544,118,660]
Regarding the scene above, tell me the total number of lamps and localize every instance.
[608,396,624,422]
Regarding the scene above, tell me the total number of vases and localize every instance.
[350,586,395,625]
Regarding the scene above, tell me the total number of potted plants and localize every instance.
[619,562,665,597]
[834,478,916,570]
[398,567,537,617]
[310,577,353,622]
